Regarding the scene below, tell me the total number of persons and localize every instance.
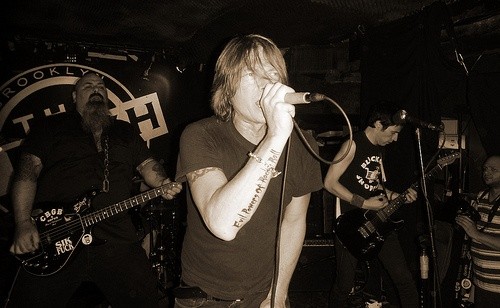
[7,70,183,308]
[452,153,500,308]
[173,34,326,308]
[321,106,421,308]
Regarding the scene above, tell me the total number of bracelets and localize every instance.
[248,152,282,179]
[350,192,366,209]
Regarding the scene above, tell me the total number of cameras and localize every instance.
[454,201,481,222]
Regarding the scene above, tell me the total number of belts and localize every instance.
[173,276,247,304]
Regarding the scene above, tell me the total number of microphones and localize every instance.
[284,92,326,104]
[393,109,443,132]
